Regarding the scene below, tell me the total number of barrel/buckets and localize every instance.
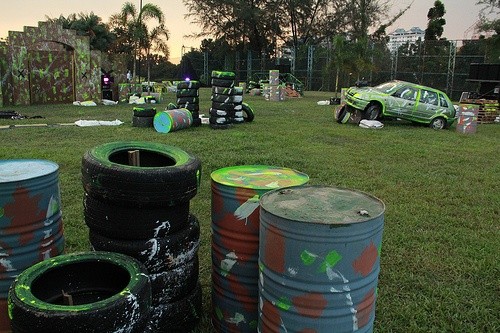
[239,83,246,94]
[456,104,480,135]
[0,159,65,333]
[255,184,387,333]
[210,165,309,333]
[118,83,142,103]
[263,70,286,102]
[250,88,260,96]
[153,108,193,133]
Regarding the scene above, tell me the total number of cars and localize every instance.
[343,79,458,130]
